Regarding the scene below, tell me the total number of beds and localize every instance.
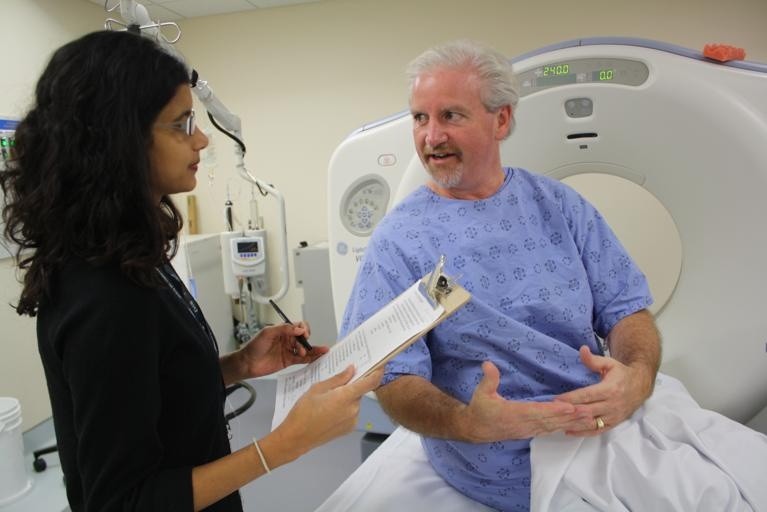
[314,425,501,512]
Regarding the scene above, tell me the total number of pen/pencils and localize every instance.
[269,300,312,351]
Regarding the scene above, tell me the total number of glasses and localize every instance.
[150,110,197,137]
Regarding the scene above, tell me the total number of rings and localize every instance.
[595,416,607,431]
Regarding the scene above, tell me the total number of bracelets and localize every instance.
[251,438,272,473]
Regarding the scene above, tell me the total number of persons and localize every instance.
[341,42,662,511]
[1,31,386,512]
